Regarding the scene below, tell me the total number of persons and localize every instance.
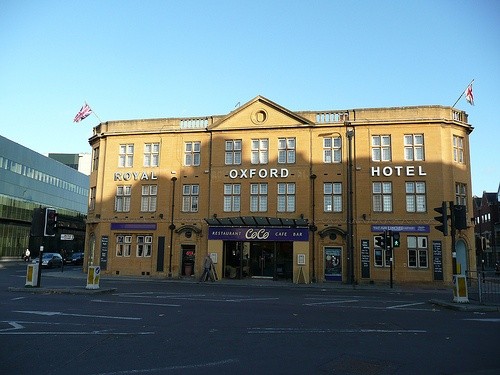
[203,254,212,281]
[24,248,30,262]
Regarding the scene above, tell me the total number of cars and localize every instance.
[64,252,85,266]
[32,253,63,269]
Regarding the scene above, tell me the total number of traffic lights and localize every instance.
[379,231,387,250]
[43,208,59,237]
[392,232,401,247]
[483,238,490,251]
[434,202,449,236]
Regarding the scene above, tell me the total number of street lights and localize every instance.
[473,197,485,283]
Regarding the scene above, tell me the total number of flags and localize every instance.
[465,84,474,105]
[73,103,93,123]
